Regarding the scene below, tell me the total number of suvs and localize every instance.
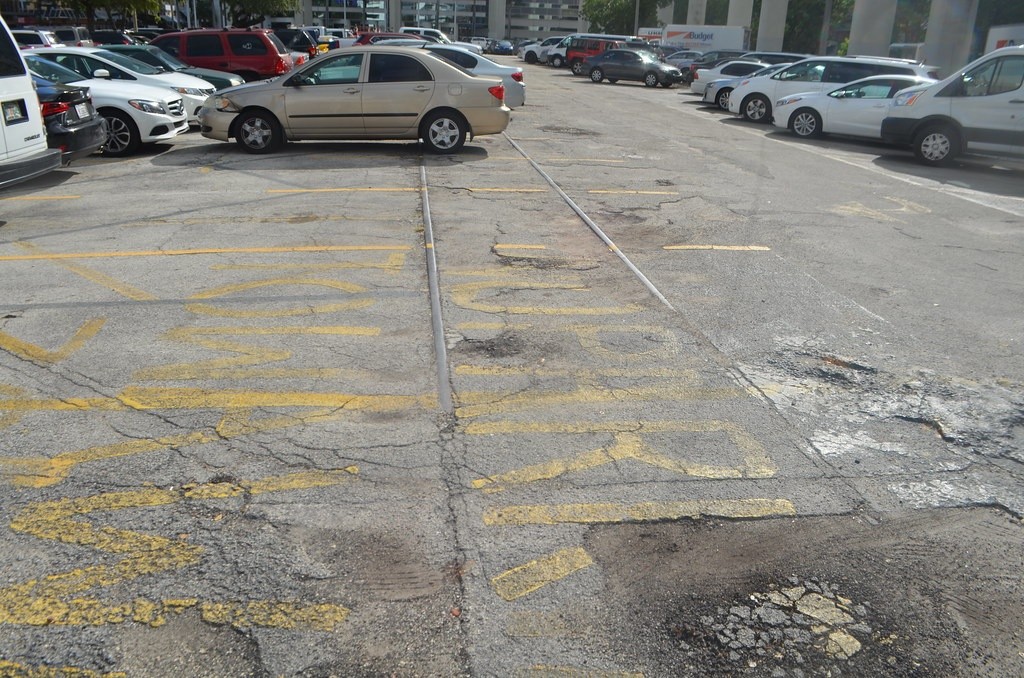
[353,27,482,56]
[521,36,567,65]
[624,42,667,63]
[9,23,160,64]
[147,26,361,91]
[565,37,631,76]
[470,37,488,53]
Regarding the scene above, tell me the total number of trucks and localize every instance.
[638,23,753,57]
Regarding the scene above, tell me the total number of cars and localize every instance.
[772,75,940,140]
[199,45,512,154]
[493,40,514,54]
[312,46,527,113]
[30,75,108,164]
[580,50,683,88]
[21,43,246,167]
[666,50,824,112]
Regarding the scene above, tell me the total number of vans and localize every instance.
[0,16,62,190]
[547,33,643,68]
[729,55,941,125]
[879,44,1024,166]
